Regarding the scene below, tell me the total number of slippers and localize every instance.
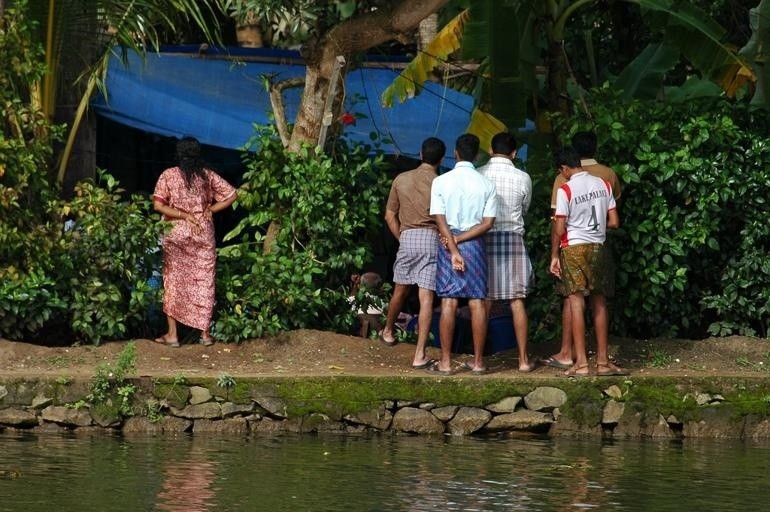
[424,364,454,376]
[413,358,436,369]
[154,336,180,348]
[377,329,396,346]
[537,351,573,369]
[457,360,490,375]
[198,335,213,346]
[519,360,536,373]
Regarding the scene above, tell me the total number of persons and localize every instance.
[475,132,536,372]
[429,133,499,373]
[539,132,621,369]
[346,273,389,314]
[349,270,360,295]
[153,136,238,348]
[378,137,446,370]
[549,145,630,376]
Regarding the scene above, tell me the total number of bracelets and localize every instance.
[179,211,181,218]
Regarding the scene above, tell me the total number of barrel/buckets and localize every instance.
[129,270,166,340]
[406,308,478,355]
[487,313,517,351]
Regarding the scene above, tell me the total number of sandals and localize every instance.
[596,361,630,375]
[564,363,590,376]
